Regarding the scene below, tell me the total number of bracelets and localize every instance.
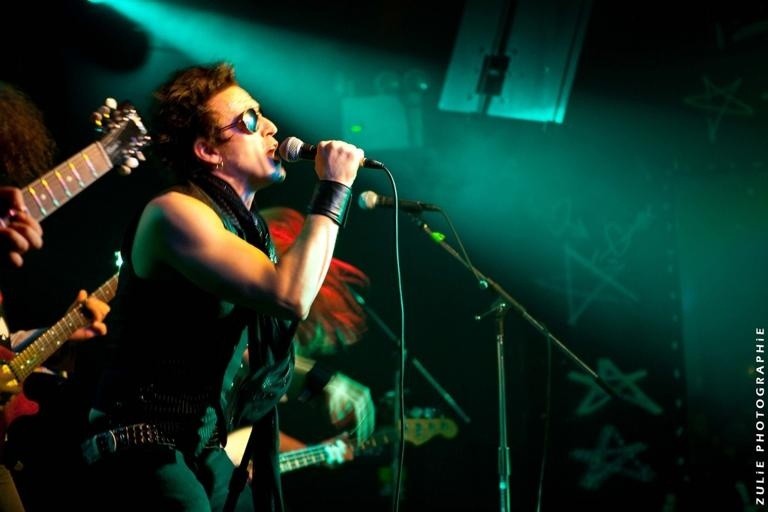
[305,361,336,391]
[305,179,352,227]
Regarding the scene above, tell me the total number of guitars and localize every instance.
[224,406,460,484]
[0,97,154,244]
[0,272,118,442]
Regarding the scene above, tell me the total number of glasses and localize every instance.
[221,109,260,133]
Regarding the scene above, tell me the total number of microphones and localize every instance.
[278,135,385,169]
[357,190,441,212]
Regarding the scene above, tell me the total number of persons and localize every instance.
[85,61,365,512]
[0,185,43,267]
[0,79,112,512]
[201,207,379,482]
